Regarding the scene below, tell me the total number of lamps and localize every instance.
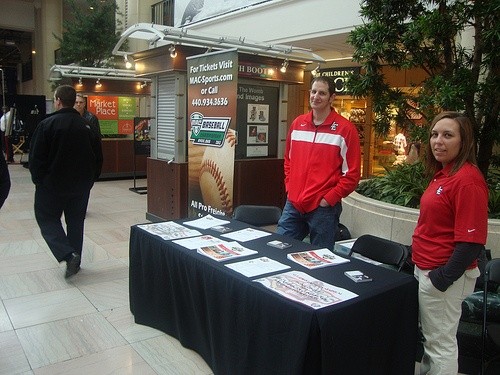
[169,45,178,58]
[123,53,132,68]
[279,58,290,73]
[311,63,320,76]
[96,78,102,87]
[78,76,83,87]
[140,81,148,88]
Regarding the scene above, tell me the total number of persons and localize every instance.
[73,93,101,133]
[28,85,103,278]
[0,104,16,163]
[393,130,407,155]
[276,76,361,249]
[31,104,40,115]
[411,111,488,375]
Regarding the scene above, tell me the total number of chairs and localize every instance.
[348,234,500,375]
[233,206,282,228]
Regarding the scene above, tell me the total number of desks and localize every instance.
[129,215,417,375]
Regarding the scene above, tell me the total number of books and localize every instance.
[287,248,351,269]
[197,241,258,262]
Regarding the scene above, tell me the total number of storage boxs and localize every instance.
[335,238,382,266]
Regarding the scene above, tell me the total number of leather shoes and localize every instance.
[65,252,81,278]
